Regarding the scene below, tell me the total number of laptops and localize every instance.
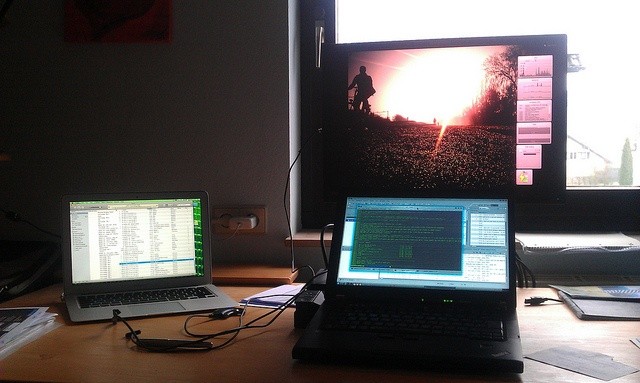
[291,192,524,372]
[60,190,239,322]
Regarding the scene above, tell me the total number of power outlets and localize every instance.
[212,206,268,236]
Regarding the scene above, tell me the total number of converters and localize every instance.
[229,216,255,229]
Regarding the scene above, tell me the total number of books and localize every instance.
[559,291,640,320]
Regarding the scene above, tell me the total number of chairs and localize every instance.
[514,255,536,287]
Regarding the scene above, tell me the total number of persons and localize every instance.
[348,64,374,118]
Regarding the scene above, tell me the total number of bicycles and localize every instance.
[347,86,373,137]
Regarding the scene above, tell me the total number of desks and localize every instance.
[0,286,639,383]
[515,229,640,284]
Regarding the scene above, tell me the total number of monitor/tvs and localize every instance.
[320,38,566,204]
